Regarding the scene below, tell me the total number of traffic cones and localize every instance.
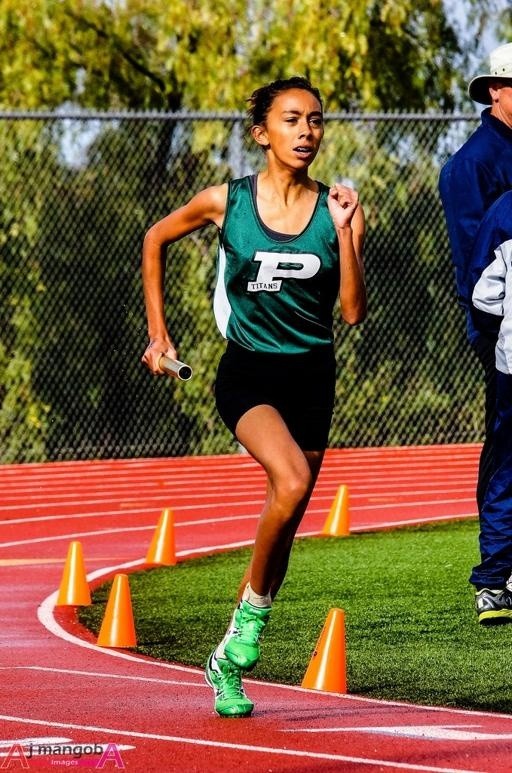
[297,607,352,693]
[93,572,135,648]
[146,508,178,566]
[319,485,357,535]
[59,539,93,610]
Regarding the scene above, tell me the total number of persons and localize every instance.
[437,41,512,376]
[139,74,371,721]
[469,236,512,626]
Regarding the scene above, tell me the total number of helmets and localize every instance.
[468,41,512,104]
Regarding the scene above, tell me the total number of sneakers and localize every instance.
[204,650,255,718]
[225,600,274,669]
[474,588,512,626]
